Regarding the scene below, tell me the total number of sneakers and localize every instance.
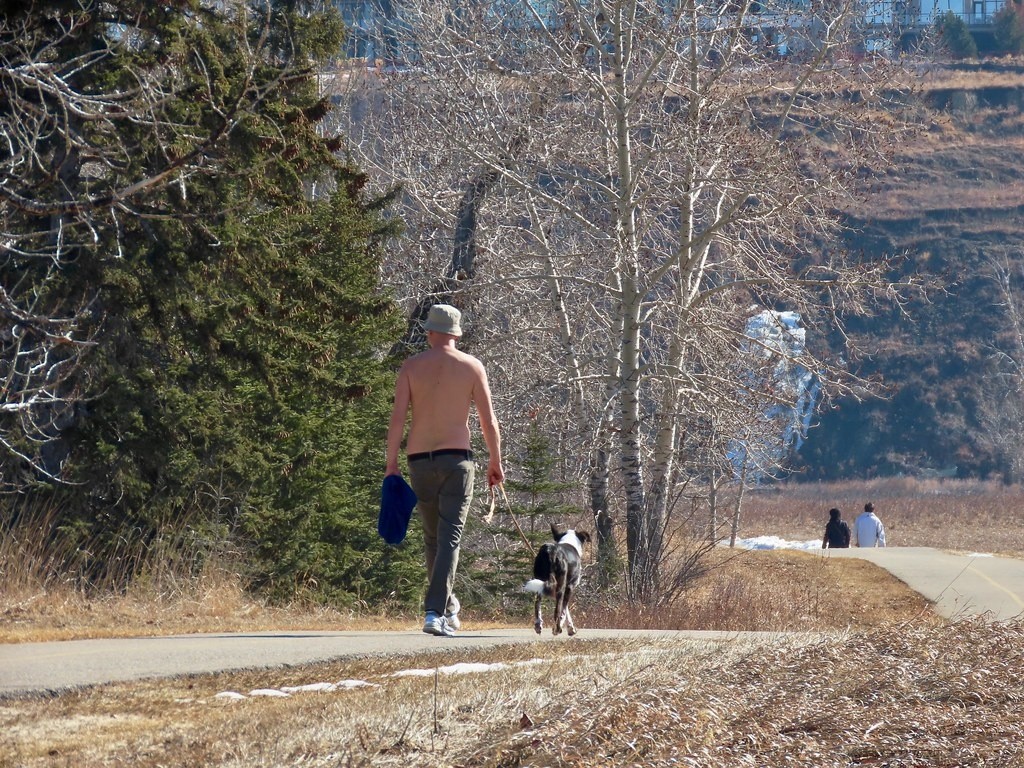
[447,614,460,630]
[423,610,455,636]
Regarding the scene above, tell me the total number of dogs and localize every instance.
[520,522,592,636]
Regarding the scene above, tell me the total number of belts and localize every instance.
[408,449,473,461]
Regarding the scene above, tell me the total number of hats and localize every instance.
[424,304,463,336]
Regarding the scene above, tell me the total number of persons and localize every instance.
[853,502,886,548]
[383,304,505,635]
[822,508,850,549]
